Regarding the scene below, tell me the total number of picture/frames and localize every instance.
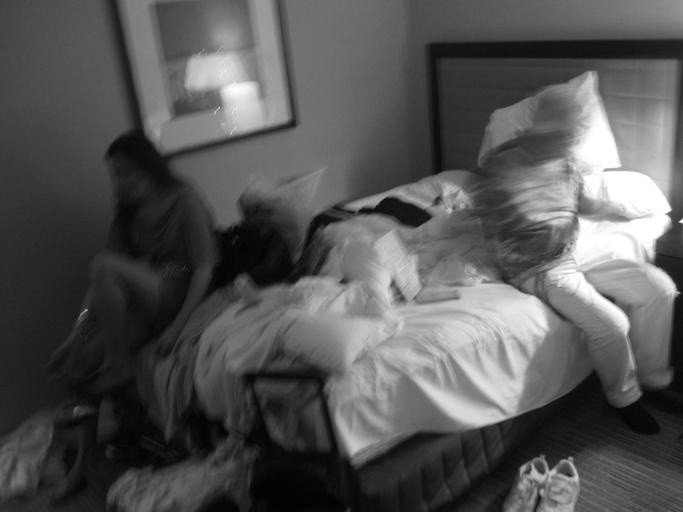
[113,1,299,159]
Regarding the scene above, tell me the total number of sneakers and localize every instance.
[501,453,581,511]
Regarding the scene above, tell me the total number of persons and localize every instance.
[475,83,682,437]
[40,125,222,488]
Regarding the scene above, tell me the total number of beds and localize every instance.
[105,38,682,511]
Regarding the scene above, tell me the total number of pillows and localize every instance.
[475,71,672,220]
